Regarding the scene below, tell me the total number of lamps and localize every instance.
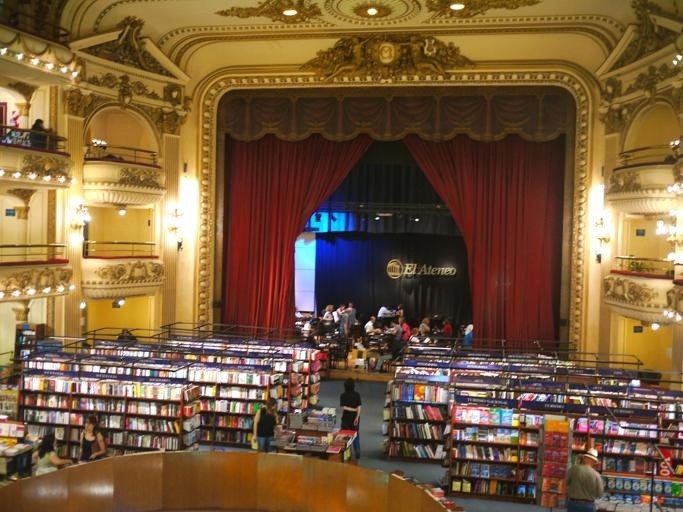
[118,206,126,217]
[112,297,126,308]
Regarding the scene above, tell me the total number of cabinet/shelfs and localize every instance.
[0,323,358,488]
[382,328,681,512]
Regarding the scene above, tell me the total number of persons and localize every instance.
[31,119,50,146]
[319,302,454,362]
[8,111,20,133]
[340,378,362,461]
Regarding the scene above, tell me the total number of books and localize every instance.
[275,406,358,463]
[1,337,321,488]
[381,344,682,511]
